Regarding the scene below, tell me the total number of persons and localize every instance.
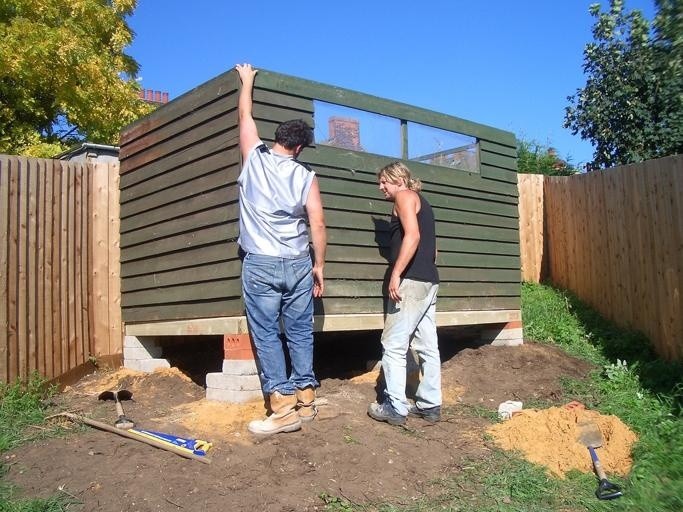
[368,162,441,424]
[236,63,326,434]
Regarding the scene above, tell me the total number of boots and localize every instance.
[295,384,317,423]
[247,390,301,436]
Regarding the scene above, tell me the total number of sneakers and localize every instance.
[411,405,440,423]
[367,397,406,425]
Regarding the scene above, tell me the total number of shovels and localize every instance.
[577,421,623,500]
[98,389,134,429]
